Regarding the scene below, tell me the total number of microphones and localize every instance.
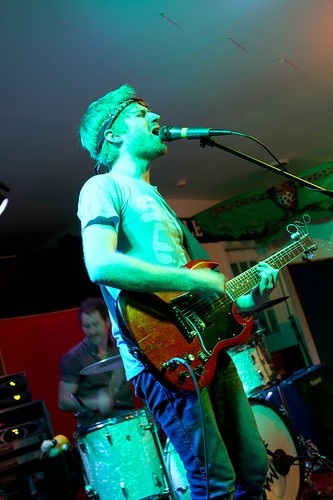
[158,125,232,141]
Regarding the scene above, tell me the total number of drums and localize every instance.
[72,409,173,500]
[164,398,303,500]
[227,341,281,398]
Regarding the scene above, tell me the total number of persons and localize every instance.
[77,84,270,499]
[58,299,134,414]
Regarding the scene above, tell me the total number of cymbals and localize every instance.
[80,353,123,376]
[254,295,291,313]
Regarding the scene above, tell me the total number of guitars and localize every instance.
[115,213,319,397]
